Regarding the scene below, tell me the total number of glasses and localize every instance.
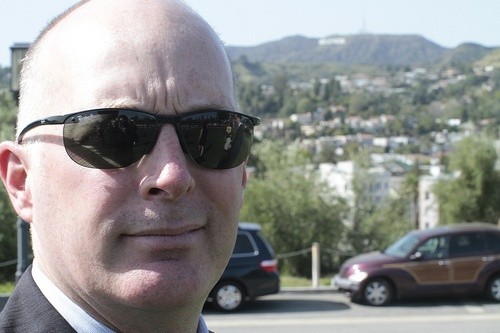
[18,107,261,170]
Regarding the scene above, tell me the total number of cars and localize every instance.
[332,222,500,307]
[200,221,281,314]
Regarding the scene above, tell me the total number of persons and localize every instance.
[0,0,261,333]
